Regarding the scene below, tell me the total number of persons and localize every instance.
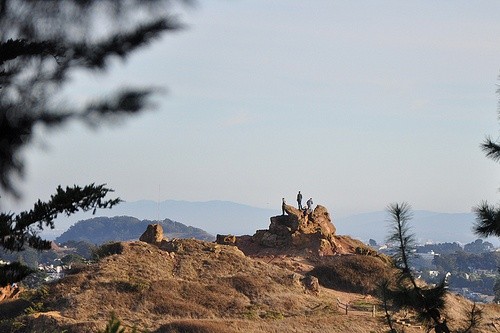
[307,197,313,209]
[281,197,285,215]
[297,190,303,210]
